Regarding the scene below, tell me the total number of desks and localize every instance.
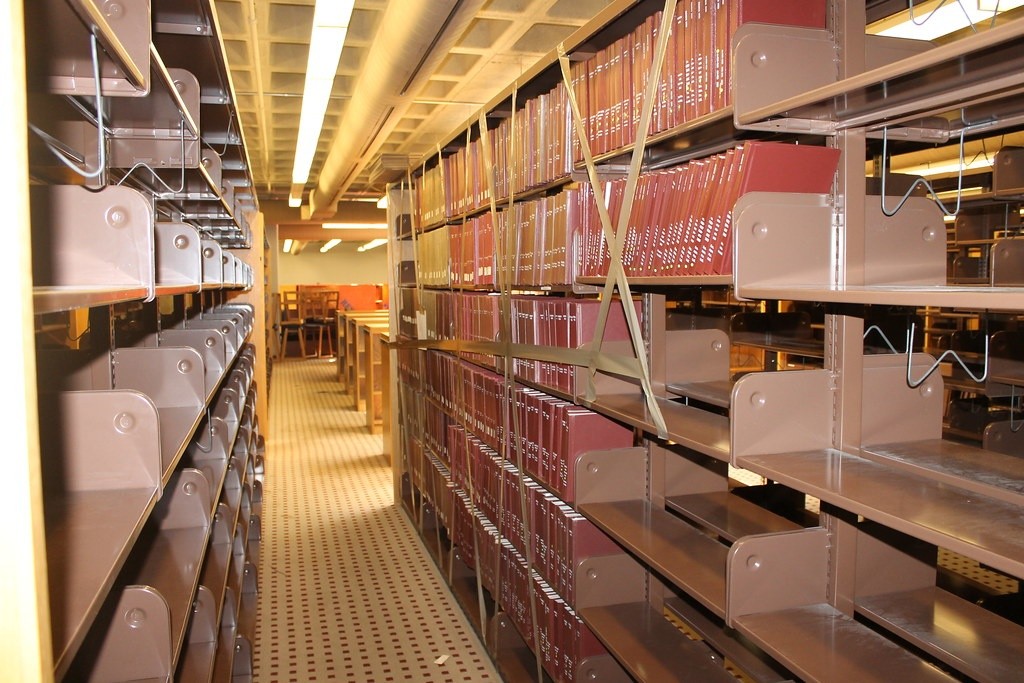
[336,310,391,467]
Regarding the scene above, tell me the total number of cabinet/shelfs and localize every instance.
[391,0,1024,683]
[1,0,273,683]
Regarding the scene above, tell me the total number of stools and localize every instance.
[280,291,339,359]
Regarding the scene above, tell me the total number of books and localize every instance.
[395,0,842,683]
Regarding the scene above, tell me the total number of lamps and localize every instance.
[864,0,1024,41]
[289,0,357,208]
[866,129,1024,181]
